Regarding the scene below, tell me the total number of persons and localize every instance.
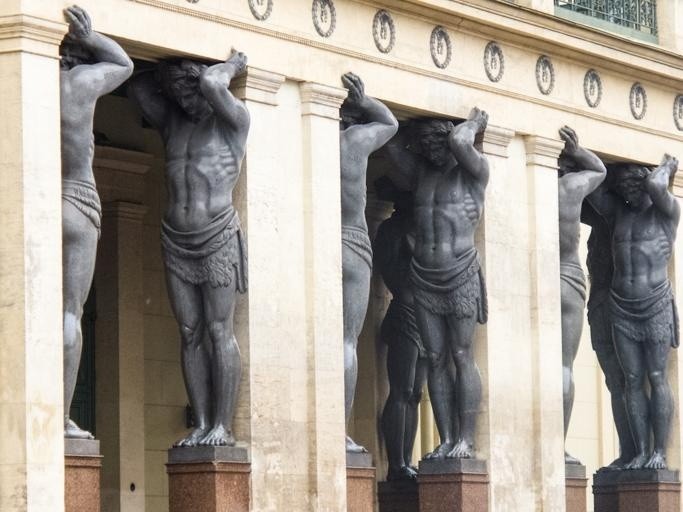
[127,48,249,449]
[61,6,135,440]
